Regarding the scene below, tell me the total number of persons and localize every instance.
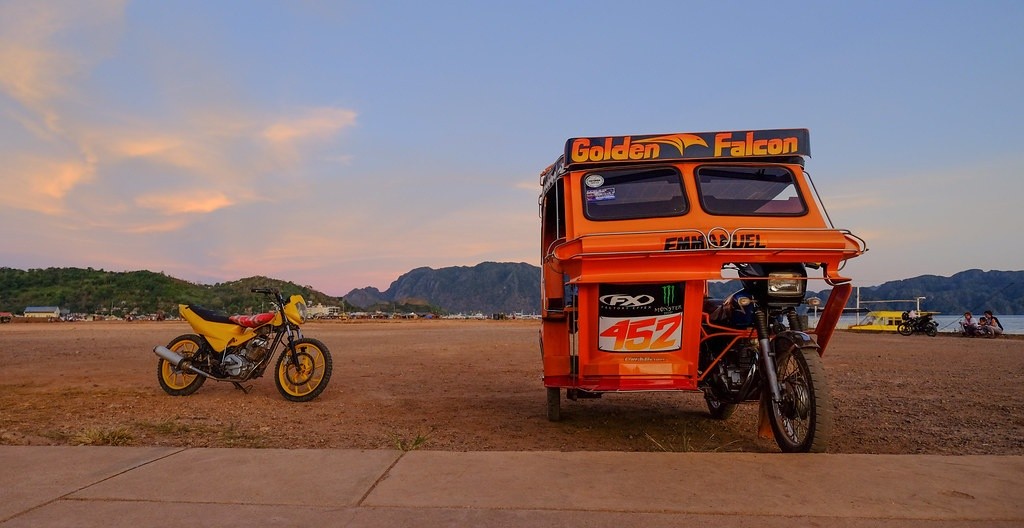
[977,310,1004,339]
[959,312,979,338]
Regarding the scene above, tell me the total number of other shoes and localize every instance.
[964,335,968,338]
[989,335,996,339]
[982,336,985,338]
[974,335,981,338]
[969,335,972,338]
[984,334,991,337]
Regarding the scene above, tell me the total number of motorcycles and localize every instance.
[152,287,332,402]
[897,310,939,337]
[699,260,822,453]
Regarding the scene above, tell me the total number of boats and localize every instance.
[849,311,940,331]
